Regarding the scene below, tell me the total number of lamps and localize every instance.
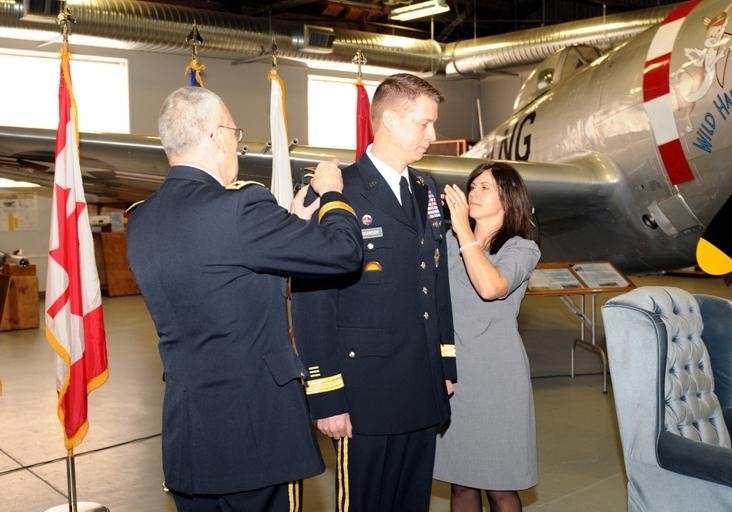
[389,0,450,22]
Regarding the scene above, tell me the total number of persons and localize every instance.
[288,71,461,510]
[426,158,542,510]
[121,78,370,512]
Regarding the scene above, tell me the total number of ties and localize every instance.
[399,178,416,229]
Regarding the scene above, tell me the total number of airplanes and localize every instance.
[1,0,732,276]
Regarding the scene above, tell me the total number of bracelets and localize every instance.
[457,240,482,253]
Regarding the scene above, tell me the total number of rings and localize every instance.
[453,200,458,206]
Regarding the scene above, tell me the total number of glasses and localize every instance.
[211,125,244,144]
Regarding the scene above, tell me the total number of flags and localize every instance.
[38,36,112,460]
[183,58,209,89]
[266,67,296,217]
[351,77,376,162]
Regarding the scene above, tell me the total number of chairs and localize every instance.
[600,285,732,511]
